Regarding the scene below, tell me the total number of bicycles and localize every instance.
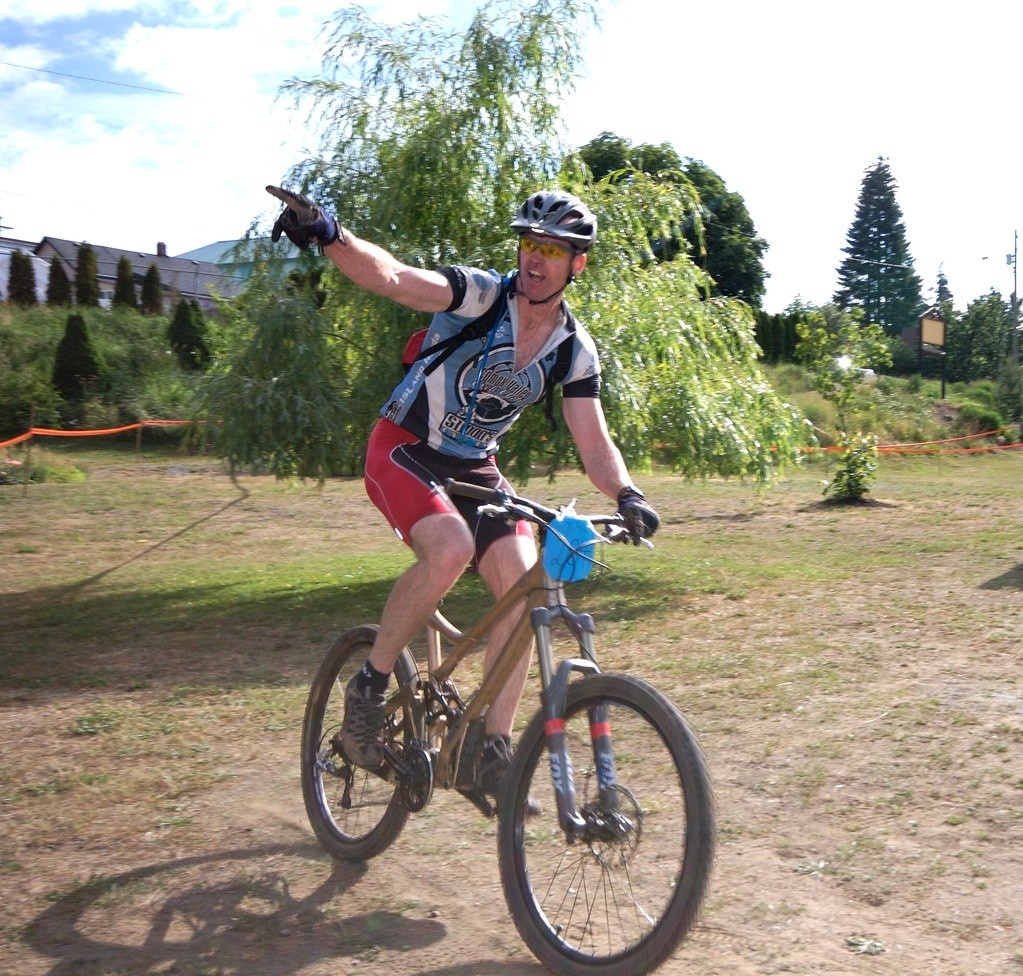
[298,477,719,976]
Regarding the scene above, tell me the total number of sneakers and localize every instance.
[340,673,385,771]
[479,743,540,817]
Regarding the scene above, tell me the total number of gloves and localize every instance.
[617,486,659,548]
[266,184,346,258]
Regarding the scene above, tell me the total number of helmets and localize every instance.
[509,189,597,254]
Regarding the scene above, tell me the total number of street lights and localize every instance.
[982,256,1017,364]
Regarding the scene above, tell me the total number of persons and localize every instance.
[267,184,659,816]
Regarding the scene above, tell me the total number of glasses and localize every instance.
[520,235,571,261]
